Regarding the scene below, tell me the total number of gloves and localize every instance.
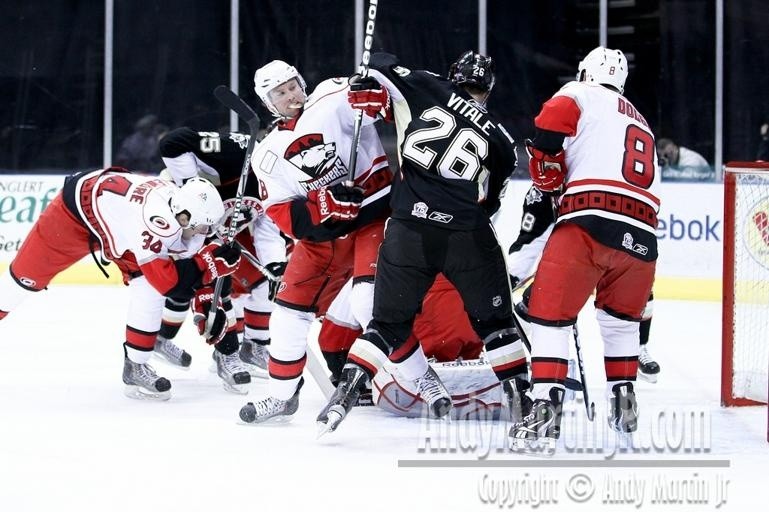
[348,76,391,117]
[528,149,567,193]
[316,183,363,223]
[195,243,242,286]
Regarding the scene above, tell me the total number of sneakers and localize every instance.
[504,377,565,438]
[413,365,452,417]
[122,335,304,422]
[638,345,659,374]
[607,382,638,432]
[317,368,367,430]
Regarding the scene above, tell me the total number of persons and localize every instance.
[155,49,528,433]
[507,185,660,376]
[0,163,241,393]
[655,137,712,179]
[508,44,663,441]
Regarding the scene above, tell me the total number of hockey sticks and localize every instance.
[205,86,260,342]
[572,323,595,421]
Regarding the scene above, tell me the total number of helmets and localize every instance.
[576,46,629,95]
[448,50,496,94]
[254,60,308,119]
[170,177,225,228]
